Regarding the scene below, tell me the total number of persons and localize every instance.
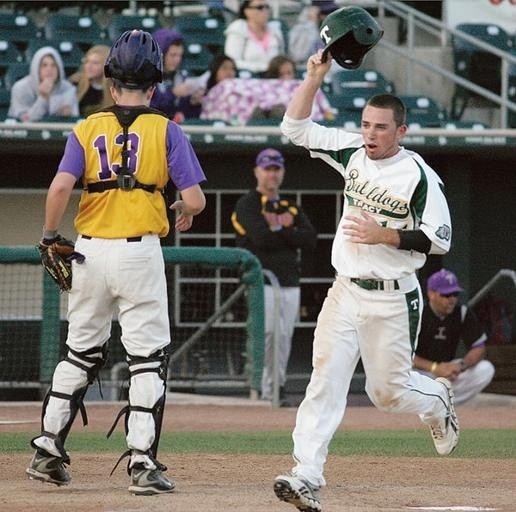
[408,269,495,407]
[273,50,459,512]
[230,149,316,401]
[27,29,206,495]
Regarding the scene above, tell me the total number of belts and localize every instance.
[350,278,400,292]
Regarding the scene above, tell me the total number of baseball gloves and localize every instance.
[37,237,85,293]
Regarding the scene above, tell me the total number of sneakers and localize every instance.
[27,449,72,486]
[427,377,461,458]
[128,467,176,495]
[272,473,323,512]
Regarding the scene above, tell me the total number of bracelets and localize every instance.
[453,358,464,373]
[431,362,437,373]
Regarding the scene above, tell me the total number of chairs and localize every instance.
[0,0,514,146]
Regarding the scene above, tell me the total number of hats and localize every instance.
[254,148,285,167]
[320,5,386,70]
[428,268,467,296]
[104,30,164,84]
